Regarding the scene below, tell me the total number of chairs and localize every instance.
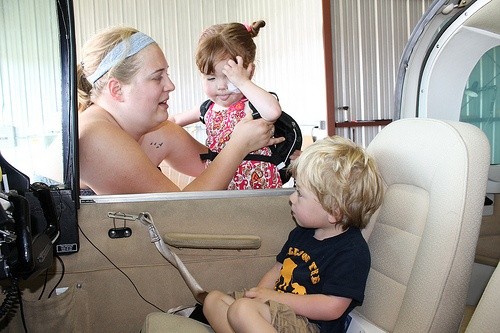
[141,118,490,333]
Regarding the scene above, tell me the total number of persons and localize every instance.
[39,27,303,195]
[166,20,281,190]
[203,135,384,333]
